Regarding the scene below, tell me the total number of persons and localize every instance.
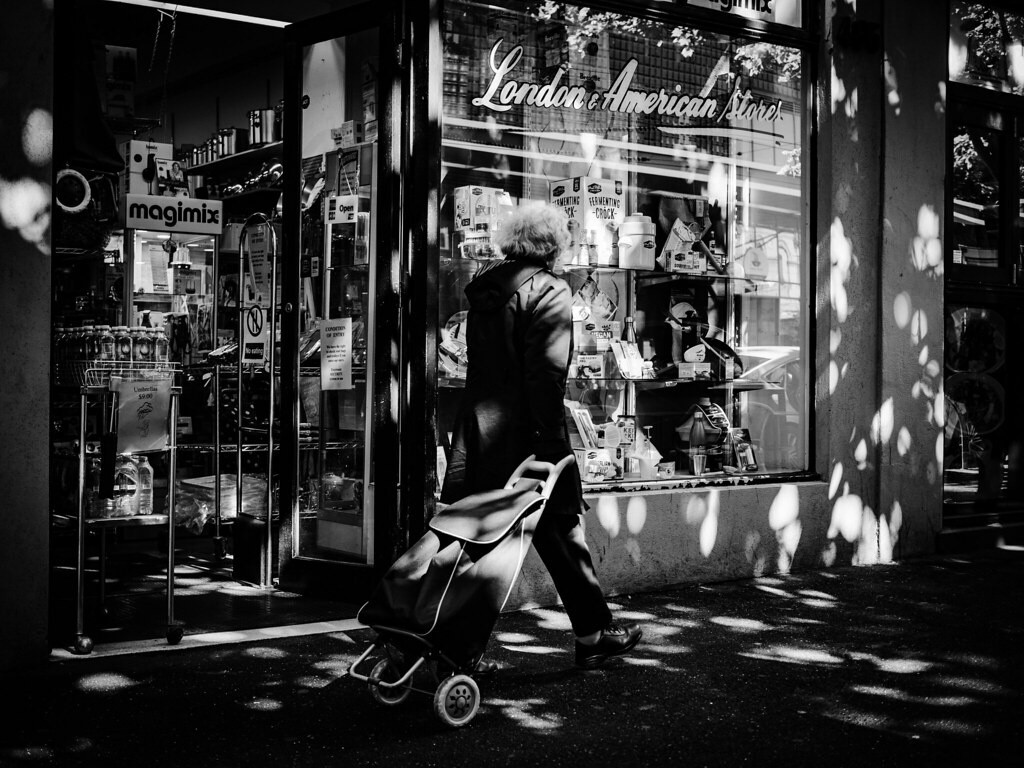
[441,202,643,680]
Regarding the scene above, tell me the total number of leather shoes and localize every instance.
[575,622,643,669]
[475,659,498,677]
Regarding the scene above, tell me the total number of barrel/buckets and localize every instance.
[182,125,243,169]
[247,109,276,144]
[617,212,656,270]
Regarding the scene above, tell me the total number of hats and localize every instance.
[665,302,698,331]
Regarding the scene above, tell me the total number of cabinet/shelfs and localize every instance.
[52,369,184,652]
[437,257,766,485]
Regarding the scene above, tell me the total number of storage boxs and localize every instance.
[550,176,626,265]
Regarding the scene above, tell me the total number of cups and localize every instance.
[693,455,707,475]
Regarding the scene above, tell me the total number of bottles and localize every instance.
[138,456,154,514]
[688,412,708,474]
[54,325,169,384]
[118,452,138,516]
[621,316,639,380]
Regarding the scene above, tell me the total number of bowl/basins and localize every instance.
[684,344,705,362]
